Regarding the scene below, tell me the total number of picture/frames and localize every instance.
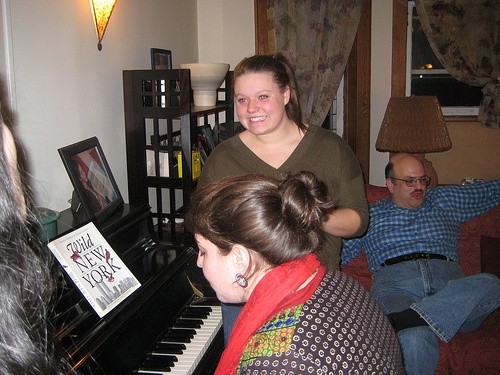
[151,47,173,70]
[58,137,124,223]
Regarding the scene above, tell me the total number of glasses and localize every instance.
[396,176,431,186]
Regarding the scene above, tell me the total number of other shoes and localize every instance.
[386,308,429,333]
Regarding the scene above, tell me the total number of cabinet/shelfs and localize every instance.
[122,68,236,249]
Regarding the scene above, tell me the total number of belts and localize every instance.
[380,252,453,267]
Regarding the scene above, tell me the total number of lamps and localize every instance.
[90,0,117,51]
[375,95,453,187]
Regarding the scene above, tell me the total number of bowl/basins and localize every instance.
[36,206,60,243]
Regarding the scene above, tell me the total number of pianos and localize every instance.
[34,201,225,375]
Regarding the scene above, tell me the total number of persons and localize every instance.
[0,100,93,375]
[196,52,370,272]
[182,169,404,375]
[72,160,105,213]
[340,152,500,375]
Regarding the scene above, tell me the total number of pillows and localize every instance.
[480,235,500,278]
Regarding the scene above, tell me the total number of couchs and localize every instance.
[341,184,500,375]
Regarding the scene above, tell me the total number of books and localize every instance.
[47,221,142,318]
